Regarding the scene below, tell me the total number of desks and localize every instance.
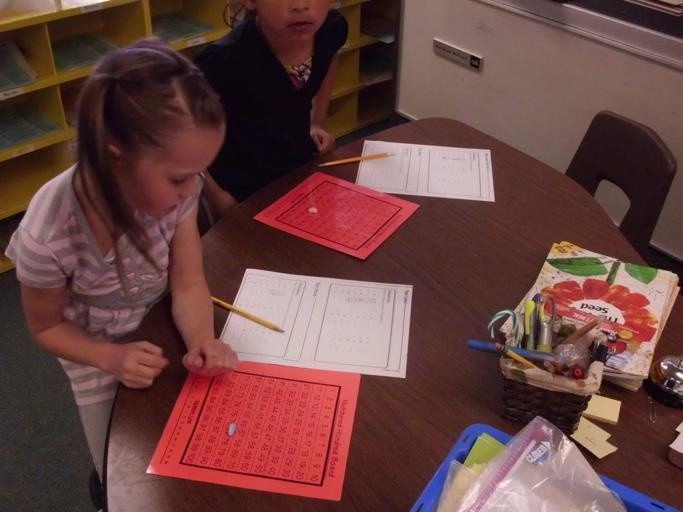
[101,116,683,512]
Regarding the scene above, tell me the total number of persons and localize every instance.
[3,35,239,488]
[191,1,348,221]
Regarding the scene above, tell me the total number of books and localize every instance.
[499,240,681,395]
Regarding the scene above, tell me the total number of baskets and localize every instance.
[498,311,608,437]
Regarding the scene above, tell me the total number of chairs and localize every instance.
[561,111,677,258]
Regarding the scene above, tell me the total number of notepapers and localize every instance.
[581,394,622,425]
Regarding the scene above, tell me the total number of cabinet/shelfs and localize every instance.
[0,0,402,278]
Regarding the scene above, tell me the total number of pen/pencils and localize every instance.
[318,152,394,167]
[467,292,597,380]
[210,295,285,333]
